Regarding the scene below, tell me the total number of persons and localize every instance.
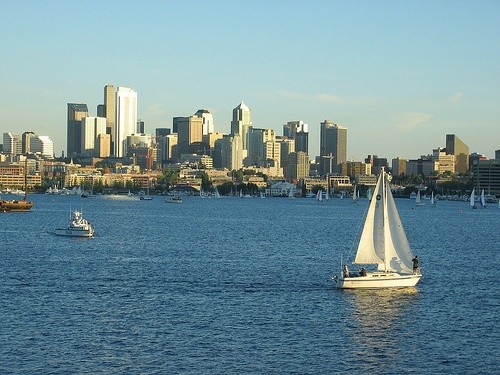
[343,265,350,278]
[411,255,419,274]
[359,267,366,277]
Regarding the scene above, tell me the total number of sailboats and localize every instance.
[240,189,266,199]
[200,187,221,199]
[326,166,422,288]
[469,188,488,209]
[315,188,330,202]
[429,191,438,207]
[413,189,425,206]
[350,184,359,202]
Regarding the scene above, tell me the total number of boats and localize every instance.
[164,193,183,203]
[0,159,33,212]
[47,204,94,237]
[140,195,152,200]
